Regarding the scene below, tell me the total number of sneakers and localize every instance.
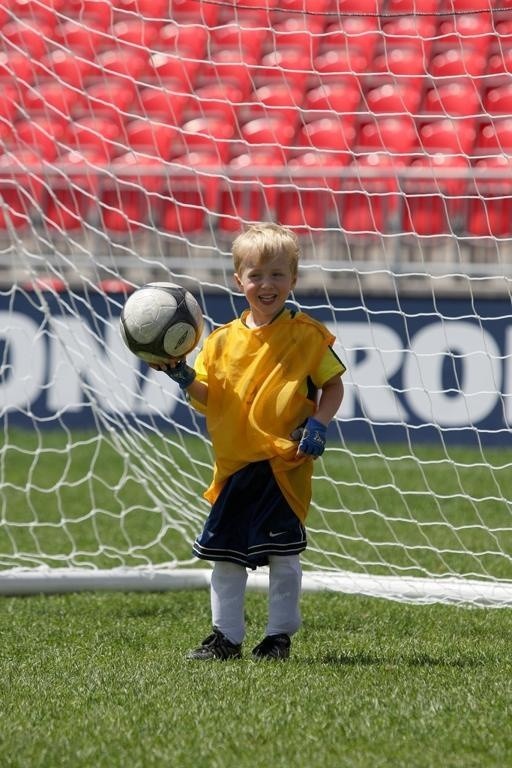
[186,625,242,660]
[250,633,291,661]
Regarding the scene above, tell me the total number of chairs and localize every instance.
[0,0,512,243]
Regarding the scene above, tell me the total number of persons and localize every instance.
[146,221,348,658]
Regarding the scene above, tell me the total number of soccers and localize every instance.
[118,282,203,360]
